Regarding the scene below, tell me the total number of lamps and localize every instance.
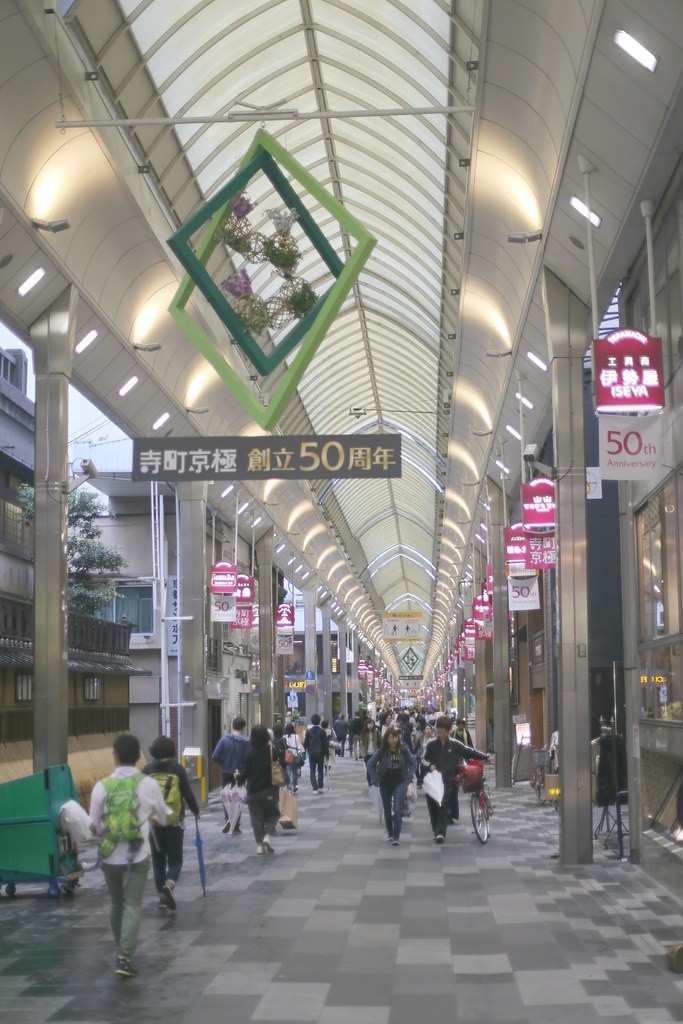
[302,551,365,626]
[505,229,544,243]
[449,574,459,579]
[454,544,467,549]
[456,520,472,525]
[132,340,163,352]
[264,500,280,506]
[31,216,73,235]
[472,430,492,436]
[463,480,479,486]
[259,390,270,407]
[288,532,300,535]
[451,562,462,566]
[187,404,212,416]
[487,349,513,358]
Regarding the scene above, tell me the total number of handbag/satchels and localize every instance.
[270,745,286,787]
[278,786,297,829]
[328,729,340,751]
[295,752,306,766]
[285,749,294,764]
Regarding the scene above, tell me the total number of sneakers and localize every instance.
[114,956,139,978]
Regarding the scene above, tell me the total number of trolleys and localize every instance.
[0,764,93,899]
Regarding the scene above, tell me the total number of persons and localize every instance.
[272,723,286,794]
[321,720,336,775]
[366,728,417,845]
[419,716,495,843]
[88,735,174,976]
[141,736,202,911]
[212,718,249,834]
[233,725,280,854]
[333,713,349,757]
[283,722,305,793]
[303,714,329,794]
[350,705,474,817]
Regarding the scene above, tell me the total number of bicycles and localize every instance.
[456,759,497,844]
[528,744,549,798]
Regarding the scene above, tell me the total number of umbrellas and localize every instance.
[219,778,247,834]
[422,768,444,807]
[368,784,384,822]
[194,816,206,897]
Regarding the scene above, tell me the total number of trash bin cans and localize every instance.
[0,764,82,898]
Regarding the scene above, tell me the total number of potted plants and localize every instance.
[289,709,308,744]
[15,478,128,649]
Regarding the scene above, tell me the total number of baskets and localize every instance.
[460,774,483,793]
[533,750,549,766]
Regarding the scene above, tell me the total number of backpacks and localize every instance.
[306,729,325,762]
[146,763,182,825]
[97,773,146,858]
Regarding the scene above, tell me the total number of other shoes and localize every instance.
[313,791,317,794]
[293,785,299,794]
[161,880,178,911]
[387,836,394,841]
[288,788,293,795]
[234,830,241,834]
[158,895,167,908]
[262,837,274,852]
[392,840,399,845]
[433,834,445,842]
[257,846,265,854]
[318,788,322,792]
[222,819,230,833]
